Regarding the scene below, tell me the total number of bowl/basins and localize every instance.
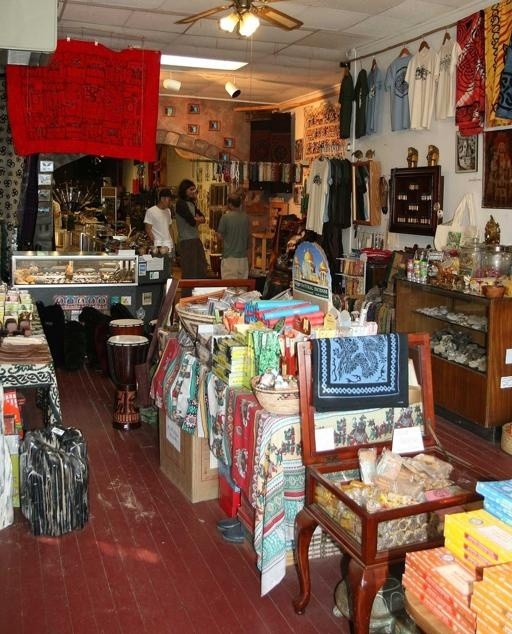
[175,304,214,348]
[250,376,299,416]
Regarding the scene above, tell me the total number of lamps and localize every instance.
[218,7,259,37]
[160,71,243,100]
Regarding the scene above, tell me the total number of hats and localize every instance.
[158,188,177,198]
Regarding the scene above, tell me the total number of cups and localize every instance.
[406,146,418,167]
[427,144,439,166]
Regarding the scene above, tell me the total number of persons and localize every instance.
[175,178,209,297]
[144,186,178,264]
[213,191,254,291]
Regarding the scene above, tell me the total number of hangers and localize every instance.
[341,27,452,78]
[314,143,349,161]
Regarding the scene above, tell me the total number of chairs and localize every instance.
[250,199,288,273]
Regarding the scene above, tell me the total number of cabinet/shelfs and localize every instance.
[335,256,365,283]
[11,256,163,324]
[390,271,512,442]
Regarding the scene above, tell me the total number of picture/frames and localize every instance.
[390,164,442,237]
[161,100,236,160]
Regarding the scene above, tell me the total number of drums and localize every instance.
[110,319,144,335]
[107,335,150,430]
[149,320,158,331]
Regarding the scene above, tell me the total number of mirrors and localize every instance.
[350,158,383,228]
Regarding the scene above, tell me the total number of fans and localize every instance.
[172,0,305,34]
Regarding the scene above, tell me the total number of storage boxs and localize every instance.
[156,407,223,505]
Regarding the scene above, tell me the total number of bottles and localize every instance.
[92,235,98,250]
[81,232,90,251]
[138,245,169,255]
[404,235,512,299]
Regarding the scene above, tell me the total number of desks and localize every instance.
[2,289,62,434]
[400,588,453,633]
[155,330,429,599]
